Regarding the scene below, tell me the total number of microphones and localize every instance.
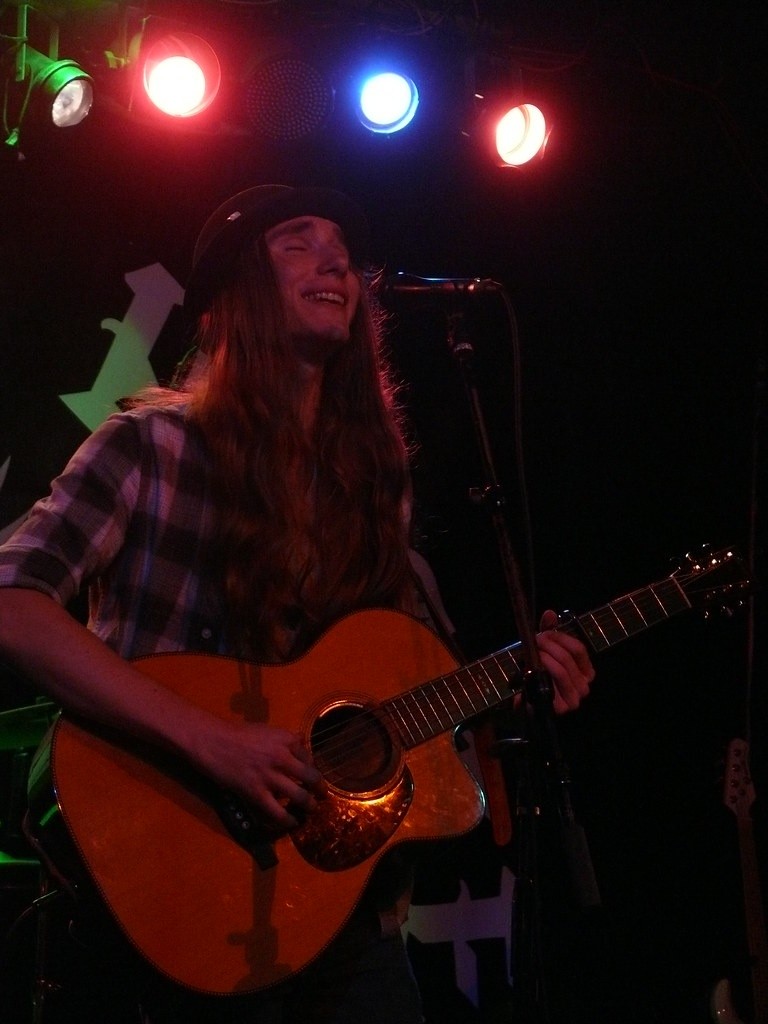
[376,271,503,310]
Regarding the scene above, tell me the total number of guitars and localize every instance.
[28,530,761,1003]
[698,734,768,1024]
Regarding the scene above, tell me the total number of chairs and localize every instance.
[0,693,59,959]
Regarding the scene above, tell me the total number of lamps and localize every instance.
[7,4,94,129]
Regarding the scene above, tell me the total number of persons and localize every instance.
[0,184,595,1024]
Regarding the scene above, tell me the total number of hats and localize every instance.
[182,185,366,321]
